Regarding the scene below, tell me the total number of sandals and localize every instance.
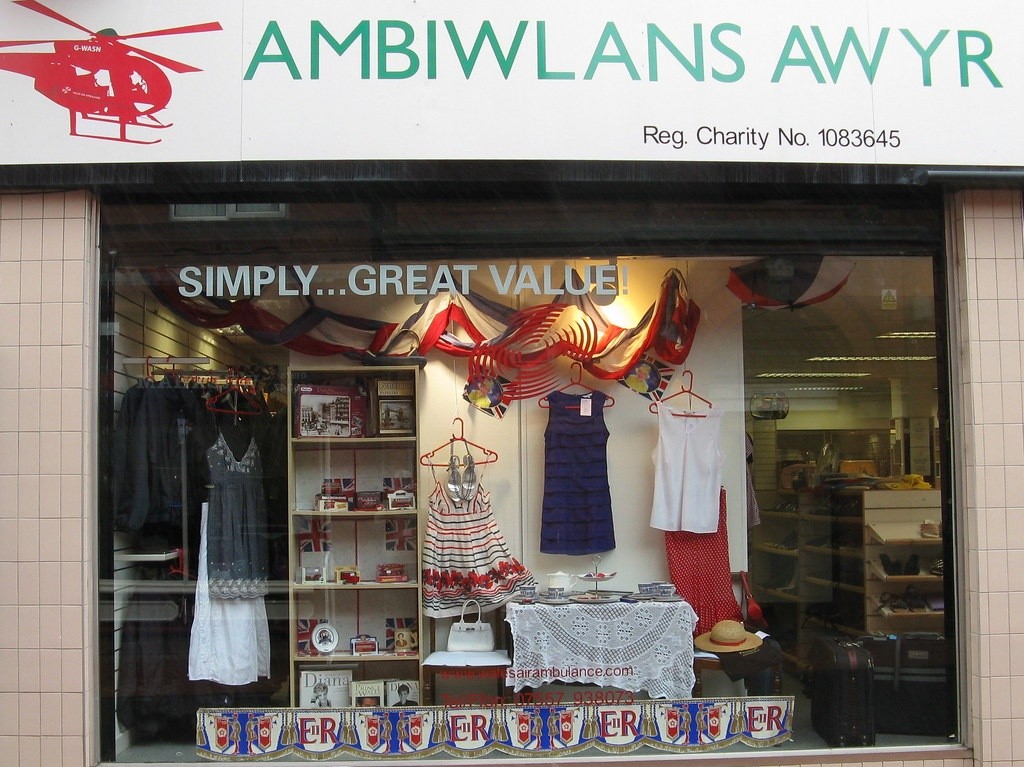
[462,455,476,501]
[445,455,463,502]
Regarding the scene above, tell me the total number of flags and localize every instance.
[385,517,418,552]
[614,350,676,403]
[299,519,333,553]
[384,617,419,650]
[298,618,330,657]
[461,362,518,421]
[323,475,417,509]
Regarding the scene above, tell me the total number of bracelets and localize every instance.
[311,699,316,704]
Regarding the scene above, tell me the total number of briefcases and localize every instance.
[812,636,876,747]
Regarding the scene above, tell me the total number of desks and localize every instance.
[421,650,512,705]
[504,589,699,698]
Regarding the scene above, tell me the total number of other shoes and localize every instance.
[763,474,944,653]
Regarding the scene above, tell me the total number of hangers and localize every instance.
[145,355,266,416]
[168,548,198,581]
[648,370,712,418]
[538,362,615,409]
[420,417,499,466]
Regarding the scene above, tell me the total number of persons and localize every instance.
[468,378,494,408]
[318,628,332,644]
[625,363,651,393]
[309,682,333,708]
[360,697,378,706]
[391,685,418,706]
[396,633,408,646]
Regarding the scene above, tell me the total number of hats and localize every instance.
[694,620,763,652]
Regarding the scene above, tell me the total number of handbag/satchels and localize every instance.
[869,633,948,734]
[740,571,769,630]
[447,600,496,652]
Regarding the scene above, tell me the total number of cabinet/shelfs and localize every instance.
[747,488,953,680]
[288,363,424,711]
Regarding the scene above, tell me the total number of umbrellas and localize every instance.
[724,254,855,315]
